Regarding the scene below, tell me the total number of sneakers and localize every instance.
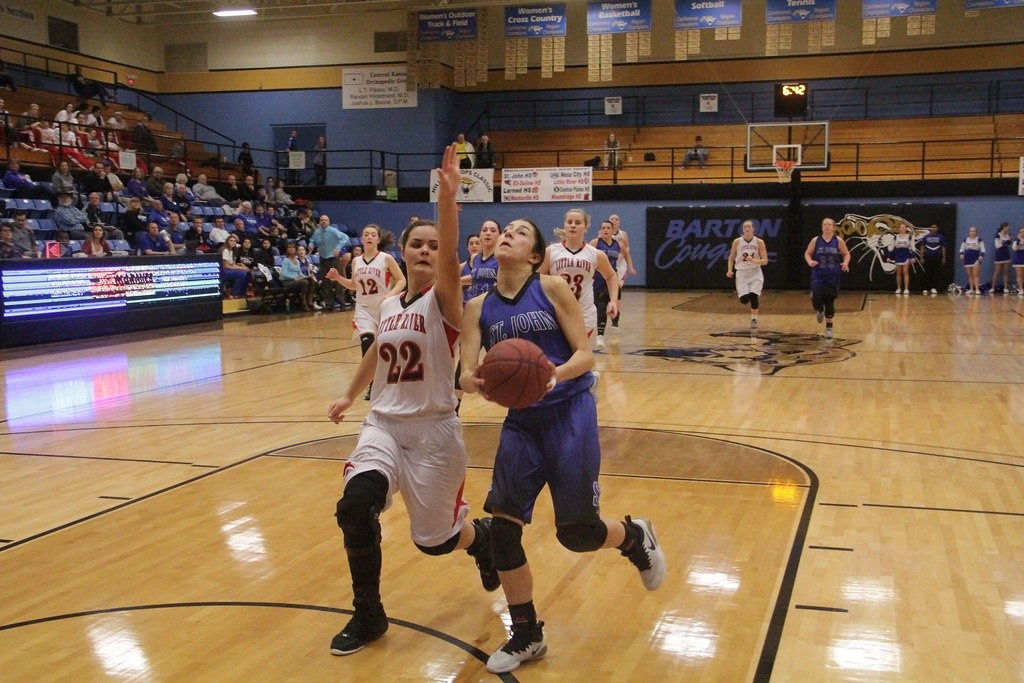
[486,620,547,673]
[466,517,502,592]
[625,515,666,591]
[330,609,388,656]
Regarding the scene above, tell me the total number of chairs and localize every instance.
[0,178,399,313]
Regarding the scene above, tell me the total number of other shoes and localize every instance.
[975,290,980,294]
[588,370,600,398]
[903,289,909,294]
[1018,289,1024,295]
[817,307,824,324]
[750,319,758,330]
[1004,289,1009,293]
[923,290,927,293]
[931,288,938,293]
[988,289,994,292]
[303,293,357,312]
[595,334,606,348]
[824,328,833,338]
[611,310,620,326]
[966,289,975,294]
[895,289,901,293]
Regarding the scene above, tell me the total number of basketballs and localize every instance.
[480,338,551,410]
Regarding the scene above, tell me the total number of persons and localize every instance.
[60,64,115,106]
[920,223,946,294]
[959,227,985,295]
[453,219,499,416]
[397,215,419,251]
[1011,227,1024,295]
[328,142,502,656]
[475,134,497,169]
[603,133,620,170]
[459,218,664,675]
[887,223,916,294]
[538,209,637,403]
[326,224,407,401]
[726,220,769,328]
[804,218,850,339]
[0,99,404,317]
[680,135,709,169]
[987,223,1013,293]
[451,133,476,169]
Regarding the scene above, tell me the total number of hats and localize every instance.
[102,159,110,165]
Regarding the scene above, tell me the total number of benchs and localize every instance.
[471,113,1024,187]
[0,83,262,184]
